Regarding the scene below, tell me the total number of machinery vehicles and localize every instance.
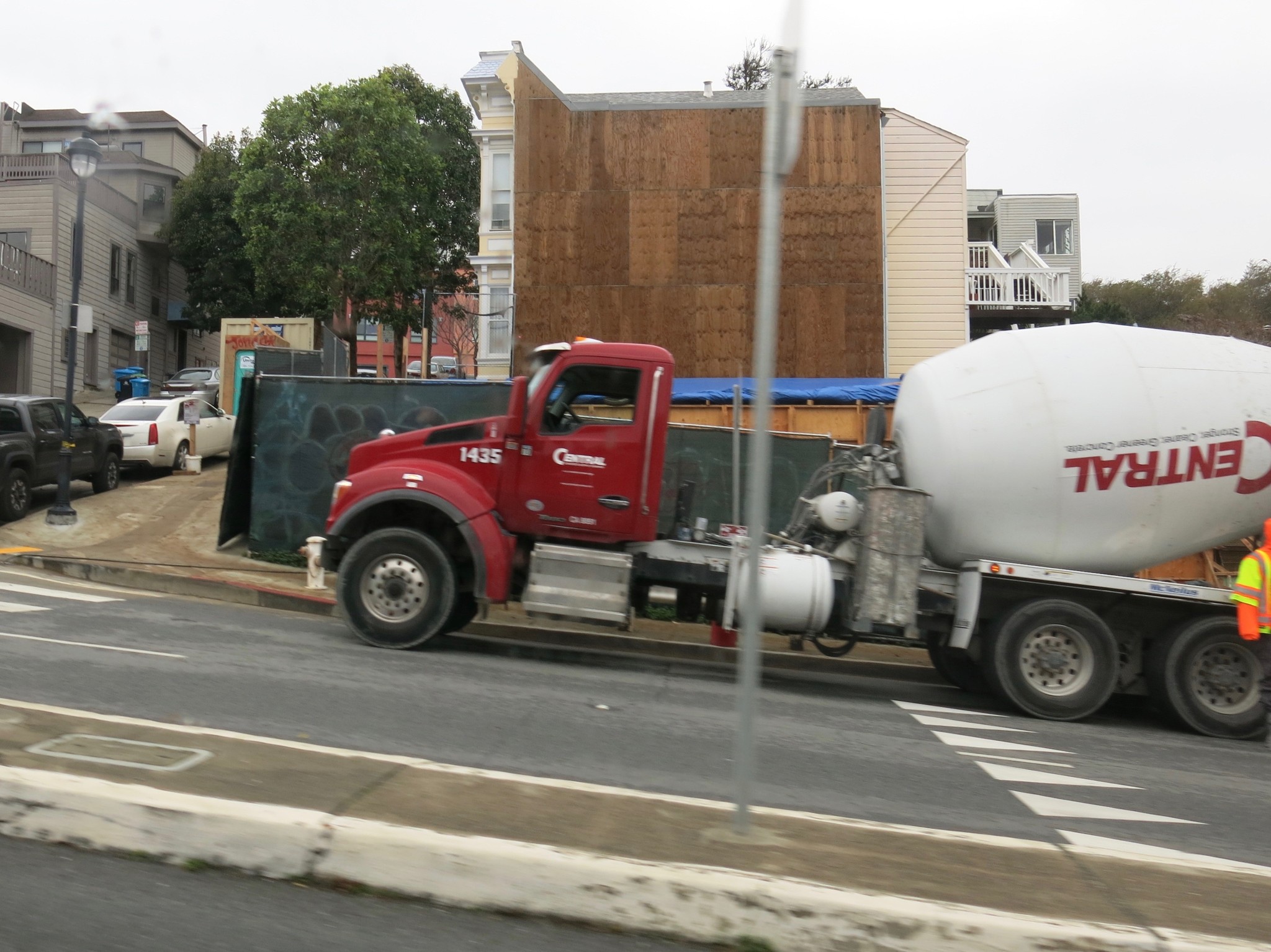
[300,321,1271,740]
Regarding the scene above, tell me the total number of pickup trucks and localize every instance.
[0,393,125,523]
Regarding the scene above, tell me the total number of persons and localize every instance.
[1228,517,1271,640]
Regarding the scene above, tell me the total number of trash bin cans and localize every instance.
[127,366,145,374]
[113,369,137,392]
[115,376,137,403]
[129,378,151,397]
[130,374,148,378]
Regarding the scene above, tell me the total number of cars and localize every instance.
[160,366,220,407]
[356,368,387,378]
[406,360,450,379]
[101,395,237,473]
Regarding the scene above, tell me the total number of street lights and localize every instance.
[44,129,103,527]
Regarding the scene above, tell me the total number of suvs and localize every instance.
[430,355,466,379]
